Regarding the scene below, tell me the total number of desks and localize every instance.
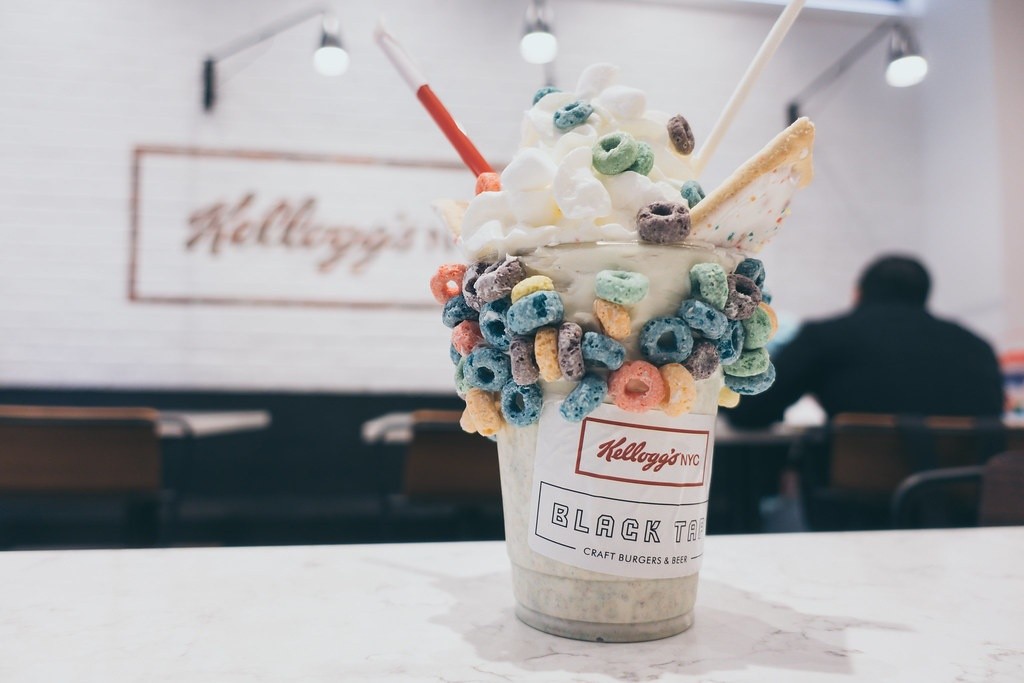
[165,412,262,439]
[709,392,825,444]
[0,526,1024,683]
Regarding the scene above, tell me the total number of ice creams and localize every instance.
[431,64,779,644]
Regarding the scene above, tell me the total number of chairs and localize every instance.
[827,415,1024,526]
[1,407,192,541]
[378,412,504,541]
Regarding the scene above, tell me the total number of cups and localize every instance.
[497,241,725,645]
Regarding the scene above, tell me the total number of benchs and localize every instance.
[0,390,824,545]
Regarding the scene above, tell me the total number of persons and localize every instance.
[716,251,1006,538]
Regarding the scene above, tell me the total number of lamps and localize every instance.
[311,17,345,77]
[521,2,556,65]
[886,26,927,92]
